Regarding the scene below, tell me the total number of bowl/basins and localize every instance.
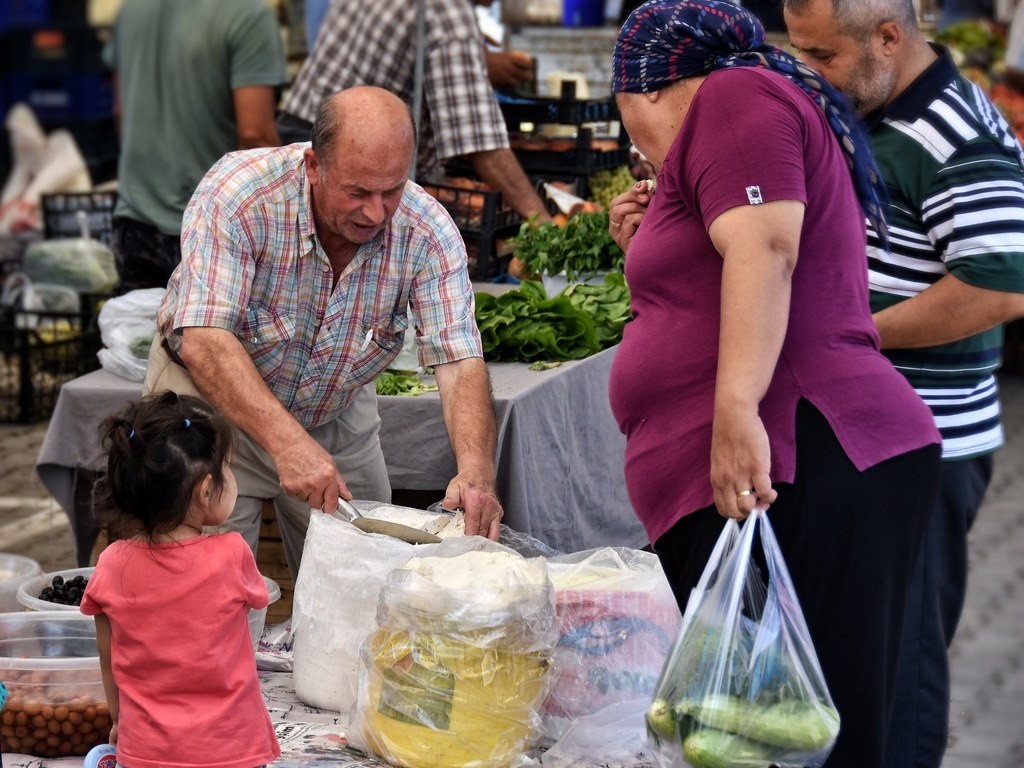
[0,549,280,759]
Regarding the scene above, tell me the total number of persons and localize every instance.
[783,0,1024,637]
[142,86,505,583]
[943,0,1024,100]
[79,389,281,768]
[608,0,949,768]
[0,0,552,296]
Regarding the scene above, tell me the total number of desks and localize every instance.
[35,281,651,555]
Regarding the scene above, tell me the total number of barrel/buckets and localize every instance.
[366,588,559,767]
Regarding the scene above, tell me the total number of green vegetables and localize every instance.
[467,269,632,371]
[371,370,440,396]
[503,205,627,281]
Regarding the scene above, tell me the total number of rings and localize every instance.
[610,220,620,228]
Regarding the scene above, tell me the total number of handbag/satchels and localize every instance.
[644,510,840,768]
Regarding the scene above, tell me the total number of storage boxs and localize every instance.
[41,189,131,279]
[0,304,103,425]
[417,74,632,285]
